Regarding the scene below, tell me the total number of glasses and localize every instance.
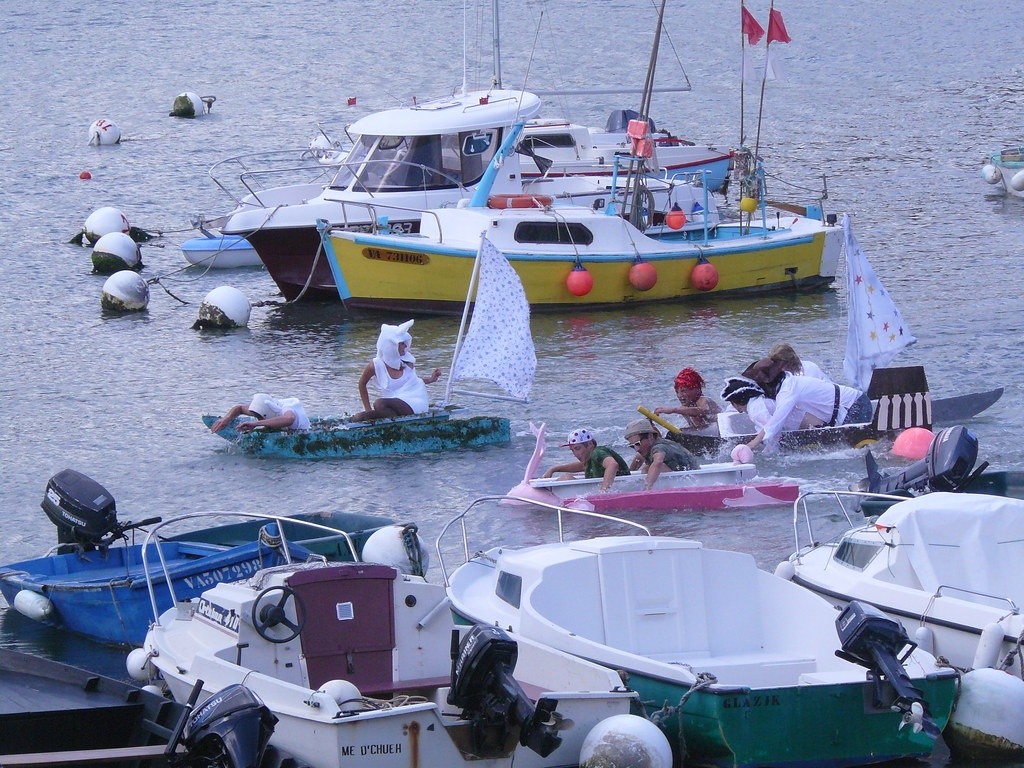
[627,435,645,448]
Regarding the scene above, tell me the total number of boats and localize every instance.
[1,470,425,649]
[773,425,1024,685]
[123,511,674,767]
[497,417,801,511]
[177,1,847,318]
[200,407,538,461]
[665,385,1005,460]
[434,495,961,768]
[980,147,1024,200]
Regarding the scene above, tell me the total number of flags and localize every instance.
[767,9,791,46]
[741,7,766,45]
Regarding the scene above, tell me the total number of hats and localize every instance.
[721,378,765,404]
[248,393,282,419]
[624,419,659,439]
[375,318,417,370]
[561,429,594,446]
[742,359,782,383]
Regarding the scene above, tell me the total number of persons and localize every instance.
[211,393,312,434]
[720,374,828,454]
[771,343,839,385]
[541,429,631,491]
[624,417,700,492]
[742,357,873,450]
[653,367,723,433]
[349,318,441,422]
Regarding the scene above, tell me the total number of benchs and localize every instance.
[0,743,189,768]
[178,541,233,557]
[674,654,817,687]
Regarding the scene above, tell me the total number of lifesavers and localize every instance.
[488,193,556,209]
